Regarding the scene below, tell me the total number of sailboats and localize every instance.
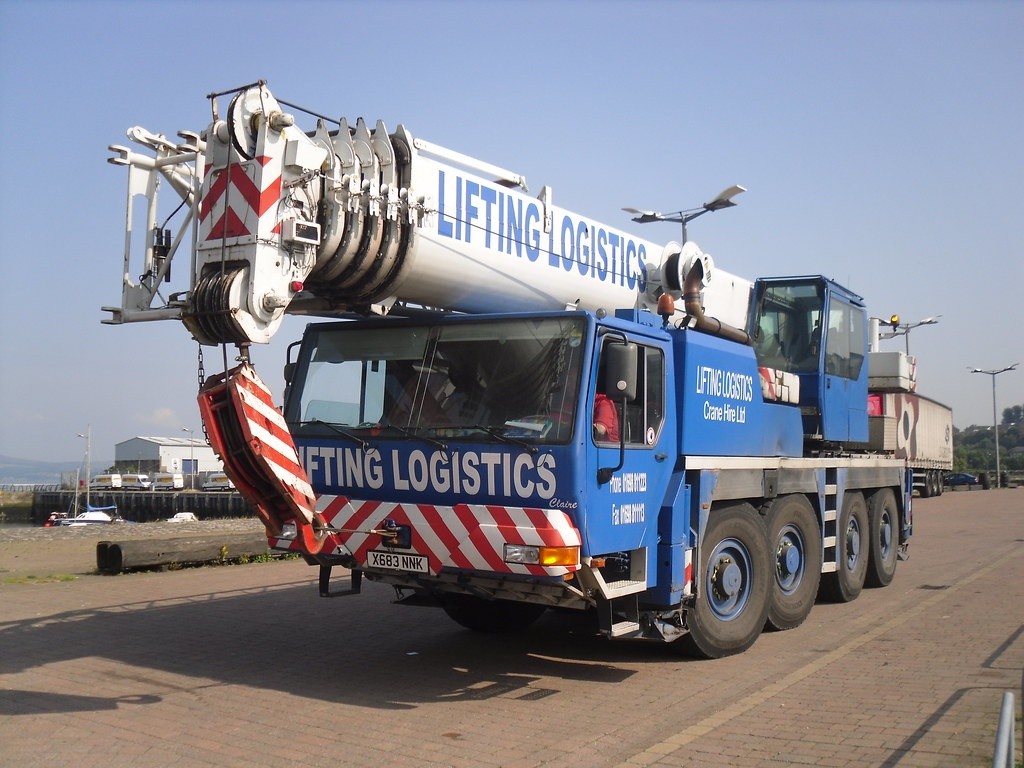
[47,423,136,528]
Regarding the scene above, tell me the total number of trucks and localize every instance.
[89,474,121,491]
[201,473,236,492]
[121,474,151,491]
[149,472,184,491]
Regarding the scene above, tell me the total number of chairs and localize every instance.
[596,371,632,443]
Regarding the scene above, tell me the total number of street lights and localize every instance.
[875,311,943,357]
[619,184,748,246]
[970,363,1021,488]
[181,428,195,490]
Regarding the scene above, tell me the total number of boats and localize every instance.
[164,511,198,523]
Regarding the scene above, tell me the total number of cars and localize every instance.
[942,471,980,486]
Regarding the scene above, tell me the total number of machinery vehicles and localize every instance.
[98,78,916,660]
